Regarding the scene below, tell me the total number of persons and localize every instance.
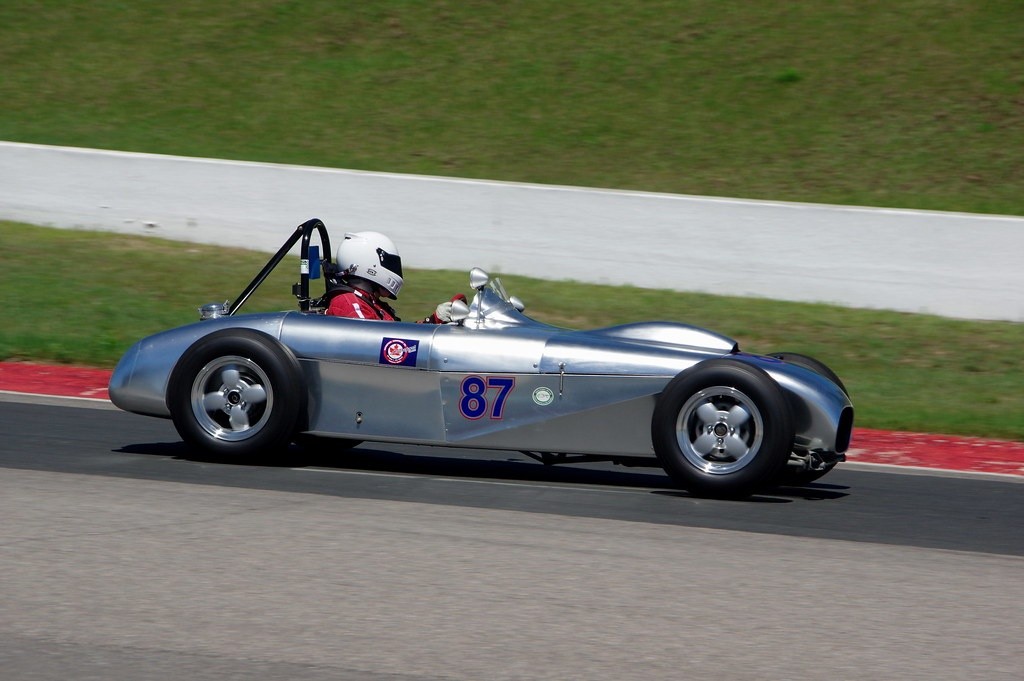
[326,231,468,324]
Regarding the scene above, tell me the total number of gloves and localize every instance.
[435,301,452,323]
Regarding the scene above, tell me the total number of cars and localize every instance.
[107,219,855,503]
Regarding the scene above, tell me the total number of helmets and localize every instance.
[335,232,404,300]
